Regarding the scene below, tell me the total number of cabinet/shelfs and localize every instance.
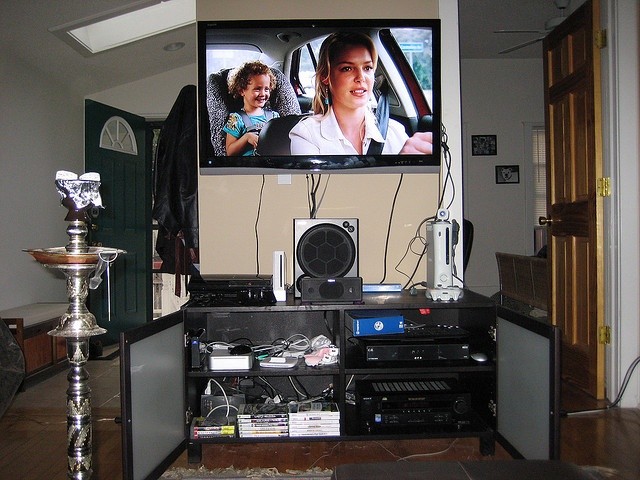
[114,288,558,480]
[0,302,70,392]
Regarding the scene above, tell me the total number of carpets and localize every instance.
[334,460,597,480]
[158,468,332,480]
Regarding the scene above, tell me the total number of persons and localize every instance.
[223,60,281,156]
[288,400,341,437]
[289,32,433,159]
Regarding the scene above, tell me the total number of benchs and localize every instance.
[495,251,549,312]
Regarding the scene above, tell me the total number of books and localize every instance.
[345,310,405,337]
[190,416,237,438]
[237,403,289,438]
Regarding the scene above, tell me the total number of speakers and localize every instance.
[301,277,362,304]
[293,218,359,299]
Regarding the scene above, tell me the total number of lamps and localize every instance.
[25,171,126,480]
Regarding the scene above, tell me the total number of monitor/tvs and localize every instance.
[197,19,441,175]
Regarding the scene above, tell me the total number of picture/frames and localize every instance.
[495,164,520,184]
[471,134,497,156]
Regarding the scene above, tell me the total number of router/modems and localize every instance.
[260,357,298,369]
